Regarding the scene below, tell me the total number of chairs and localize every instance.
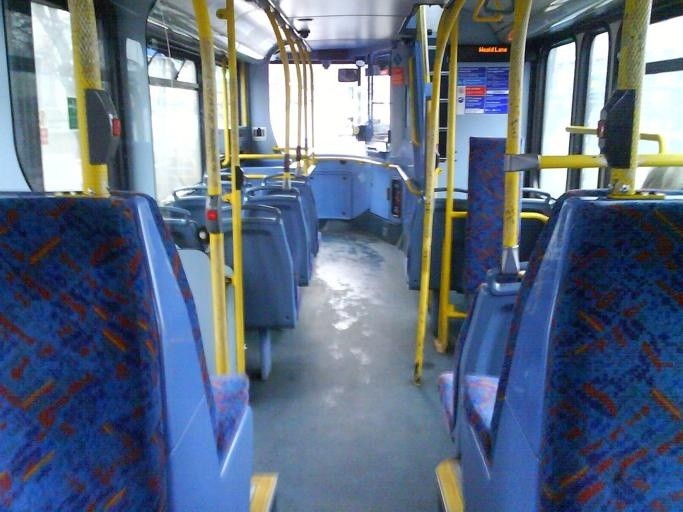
[436,261,533,460]
[453,187,683,511]
[219,204,299,330]
[460,137,530,291]
[1,192,255,511]
[171,187,206,226]
[246,185,313,286]
[202,173,256,194]
[263,172,322,256]
[157,206,205,251]
